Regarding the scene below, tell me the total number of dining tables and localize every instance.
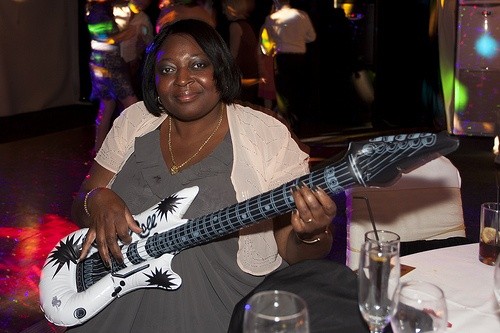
[354,243,500,333]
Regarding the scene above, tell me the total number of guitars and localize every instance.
[38,130,459,328]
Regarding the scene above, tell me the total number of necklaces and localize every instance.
[167,102,225,175]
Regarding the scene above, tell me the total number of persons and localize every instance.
[78,0,363,159]
[62,17,339,333]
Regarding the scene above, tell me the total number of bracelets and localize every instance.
[295,226,329,245]
[84,187,106,216]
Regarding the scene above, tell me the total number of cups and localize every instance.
[358,230,400,333]
[243,290,309,333]
[389,280,448,333]
[478,202,500,266]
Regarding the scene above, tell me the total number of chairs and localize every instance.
[343,154,466,274]
